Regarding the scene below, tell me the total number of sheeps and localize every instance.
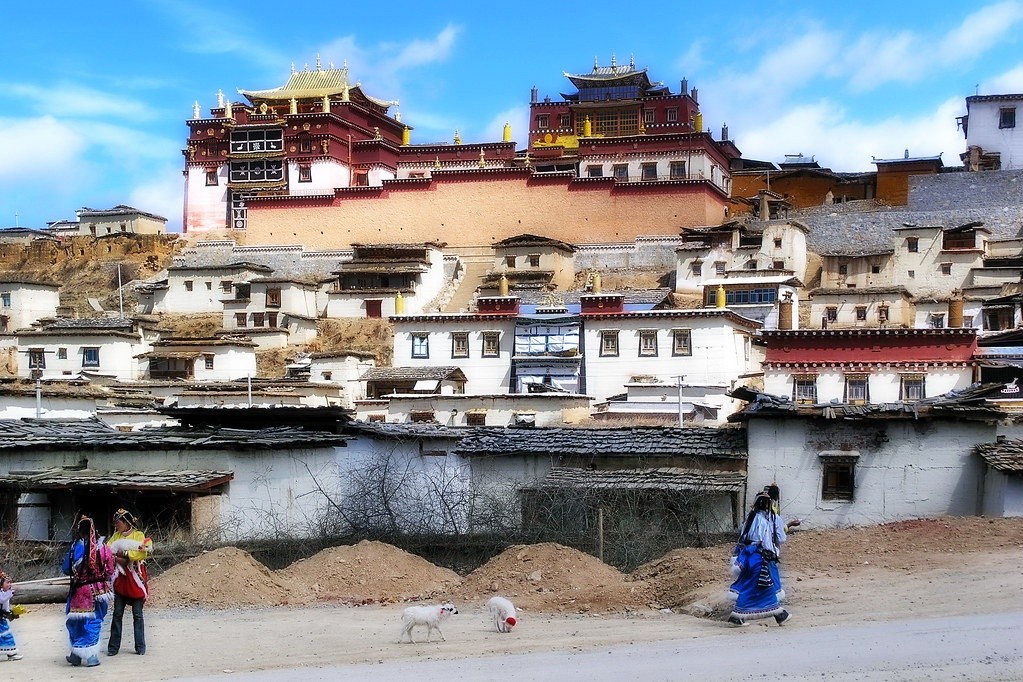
[486,597,516,633]
[110,537,152,576]
[397,604,458,644]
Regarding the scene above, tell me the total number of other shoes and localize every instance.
[65,652,81,666]
[138,651,144,655]
[108,652,114,655]
[778,613,792,626]
[728,618,751,628]
[8,655,23,661]
[87,657,100,666]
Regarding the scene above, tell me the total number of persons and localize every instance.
[0,569,23,660]
[62,515,114,667]
[729,486,792,628]
[103,509,154,657]
